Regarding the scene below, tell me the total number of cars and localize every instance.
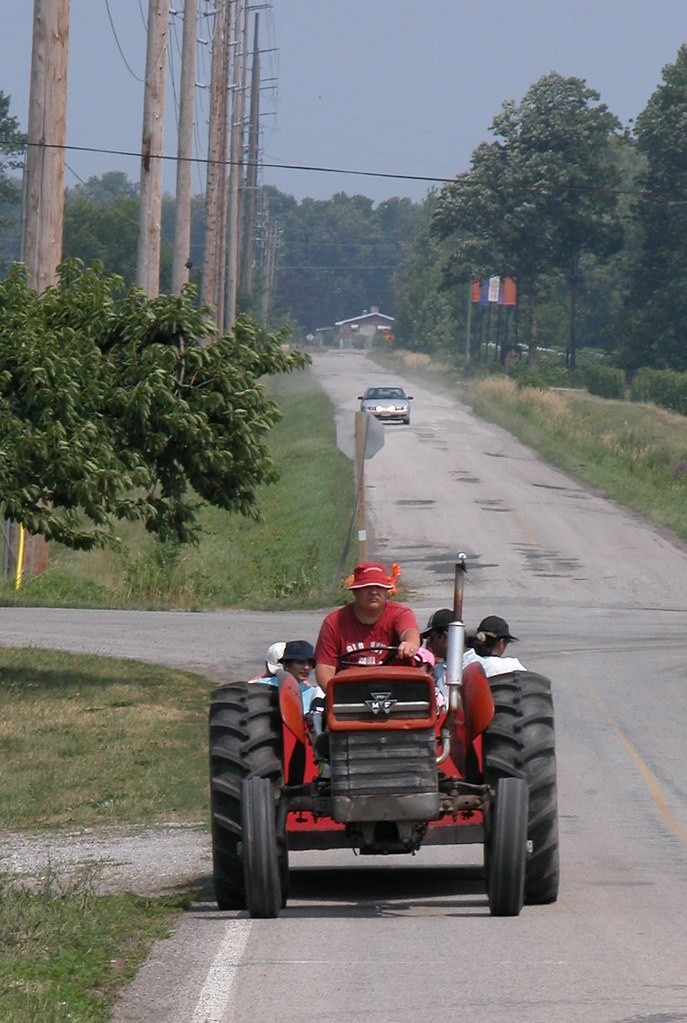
[356,386,415,425]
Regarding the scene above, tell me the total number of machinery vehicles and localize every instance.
[206,549,558,920]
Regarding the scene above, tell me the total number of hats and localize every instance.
[478,616,519,642]
[420,609,464,638]
[277,640,317,668]
[265,642,286,674]
[347,563,395,591]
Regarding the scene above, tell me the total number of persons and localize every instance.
[253,642,286,679]
[420,608,489,679]
[474,616,527,679]
[272,640,316,713]
[316,563,435,695]
